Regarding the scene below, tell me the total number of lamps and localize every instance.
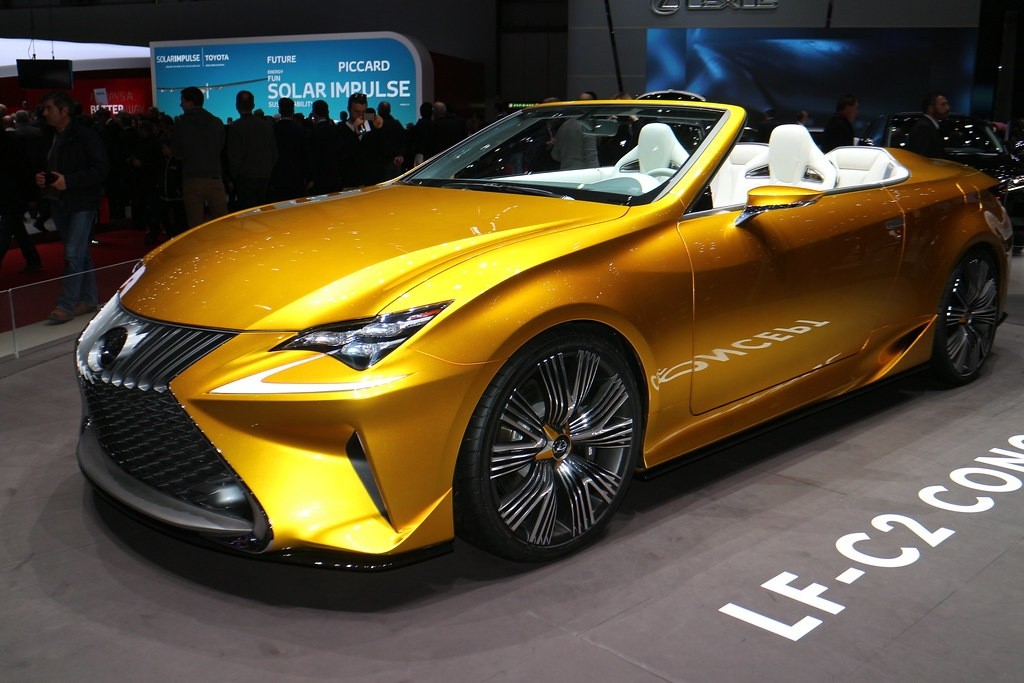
[16,6,74,90]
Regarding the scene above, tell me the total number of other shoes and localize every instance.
[19,261,40,274]
[88,239,105,246]
[47,307,74,322]
[142,232,158,245]
[70,303,99,316]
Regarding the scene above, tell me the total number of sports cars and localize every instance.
[74,99,1010,611]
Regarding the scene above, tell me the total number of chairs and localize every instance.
[710,125,908,208]
[614,122,689,181]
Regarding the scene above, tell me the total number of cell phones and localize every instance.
[42,173,55,184]
[361,113,376,121]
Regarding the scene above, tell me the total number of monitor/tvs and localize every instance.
[16,59,73,90]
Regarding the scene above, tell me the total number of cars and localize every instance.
[633,89,759,142]
[856,112,1024,249]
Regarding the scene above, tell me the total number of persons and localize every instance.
[796,111,808,126]
[507,91,643,175]
[0,86,509,278]
[822,95,873,153]
[764,110,777,132]
[910,95,962,163]
[32,90,108,320]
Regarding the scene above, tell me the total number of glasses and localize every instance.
[352,93,366,100]
